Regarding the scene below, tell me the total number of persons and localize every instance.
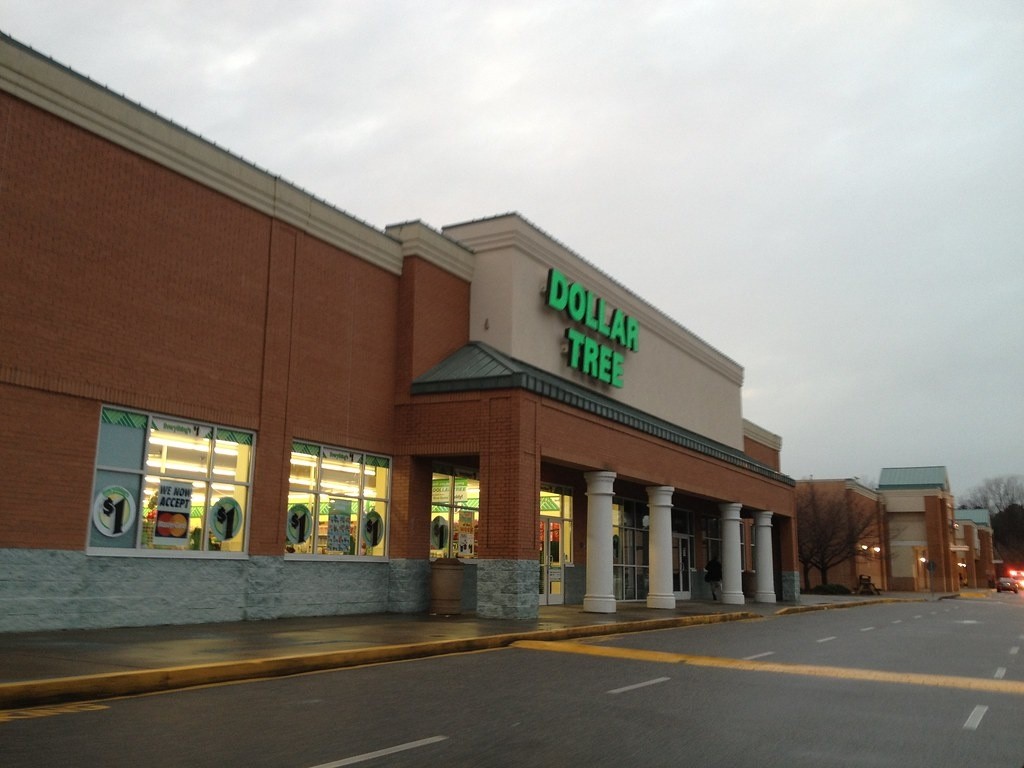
[987,574,995,589]
[148,491,158,510]
[704,555,722,600]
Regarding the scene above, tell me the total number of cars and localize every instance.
[997,578,1018,594]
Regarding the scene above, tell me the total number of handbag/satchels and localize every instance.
[705,573,712,582]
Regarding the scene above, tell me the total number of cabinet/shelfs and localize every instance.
[309,521,356,547]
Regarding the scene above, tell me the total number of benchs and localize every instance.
[858,574,885,595]
